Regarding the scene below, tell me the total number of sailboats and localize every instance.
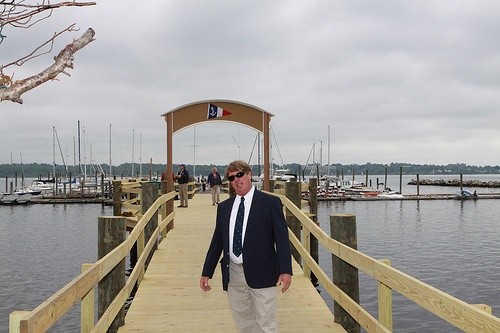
[4,119,403,203]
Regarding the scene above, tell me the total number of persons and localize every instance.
[199,160,292,333]
[207,166,223,205]
[201,176,206,191]
[175,164,190,207]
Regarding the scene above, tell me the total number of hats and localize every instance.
[178,164,185,167]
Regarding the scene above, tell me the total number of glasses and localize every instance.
[228,172,246,182]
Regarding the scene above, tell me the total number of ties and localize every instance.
[233,196,246,258]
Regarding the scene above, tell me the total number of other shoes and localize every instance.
[178,206,183,207]
[183,206,188,207]
[212,203,215,205]
[216,202,219,204]
